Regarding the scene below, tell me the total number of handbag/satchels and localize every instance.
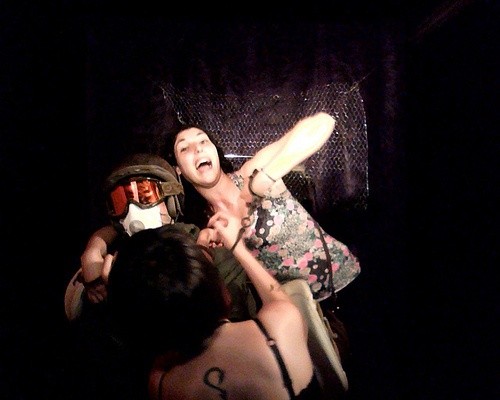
[325,302,361,362]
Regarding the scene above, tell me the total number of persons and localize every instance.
[82,114,361,304]
[107,224,324,400]
[107,156,263,323]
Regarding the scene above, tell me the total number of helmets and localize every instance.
[107,153,184,232]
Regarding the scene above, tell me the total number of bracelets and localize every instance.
[231,230,242,251]
[261,168,276,182]
[198,245,214,259]
[248,168,265,200]
[83,276,102,287]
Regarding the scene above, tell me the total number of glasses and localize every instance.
[107,177,183,220]
